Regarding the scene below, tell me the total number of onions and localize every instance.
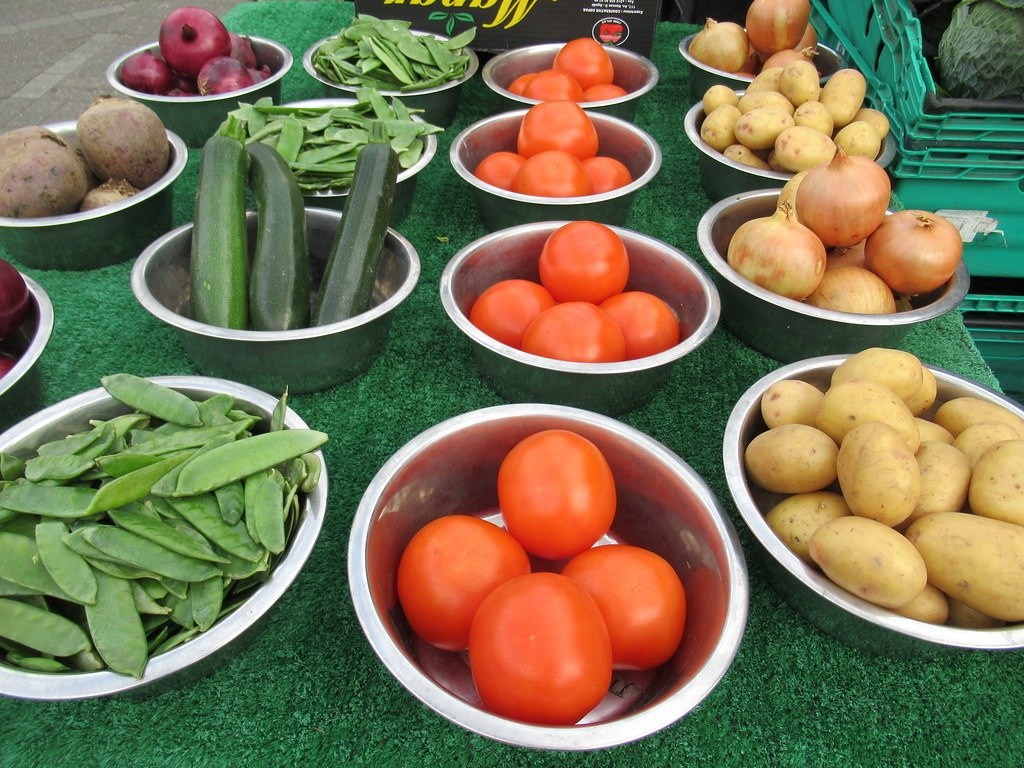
[727,147,962,315]
[688,0,822,81]
[0,255,32,377]
[119,7,271,96]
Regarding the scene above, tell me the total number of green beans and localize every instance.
[230,85,446,190]
[313,12,477,92]
[0,373,331,683]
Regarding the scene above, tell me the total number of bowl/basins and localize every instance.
[722,353,1023,663]
[482,42,659,123]
[302,29,479,132]
[449,108,662,232]
[130,207,421,398]
[696,187,971,365]
[0,270,55,396]
[679,29,849,106]
[276,98,438,226]
[105,35,294,148]
[684,89,896,204]
[0,376,328,701]
[0,120,188,270]
[345,401,751,752]
[439,221,720,417]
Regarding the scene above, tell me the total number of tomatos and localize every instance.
[474,98,633,199]
[398,430,685,726]
[466,220,681,364]
[507,36,630,104]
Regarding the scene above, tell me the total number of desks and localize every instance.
[0,0,1024,768]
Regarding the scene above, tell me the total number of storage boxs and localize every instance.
[961,290,1024,404]
[802,0,1024,278]
[820,0,1024,148]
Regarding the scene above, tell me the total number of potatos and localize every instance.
[0,93,169,217]
[699,62,889,173]
[744,347,1024,626]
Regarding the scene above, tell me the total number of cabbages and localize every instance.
[918,0,1024,102]
